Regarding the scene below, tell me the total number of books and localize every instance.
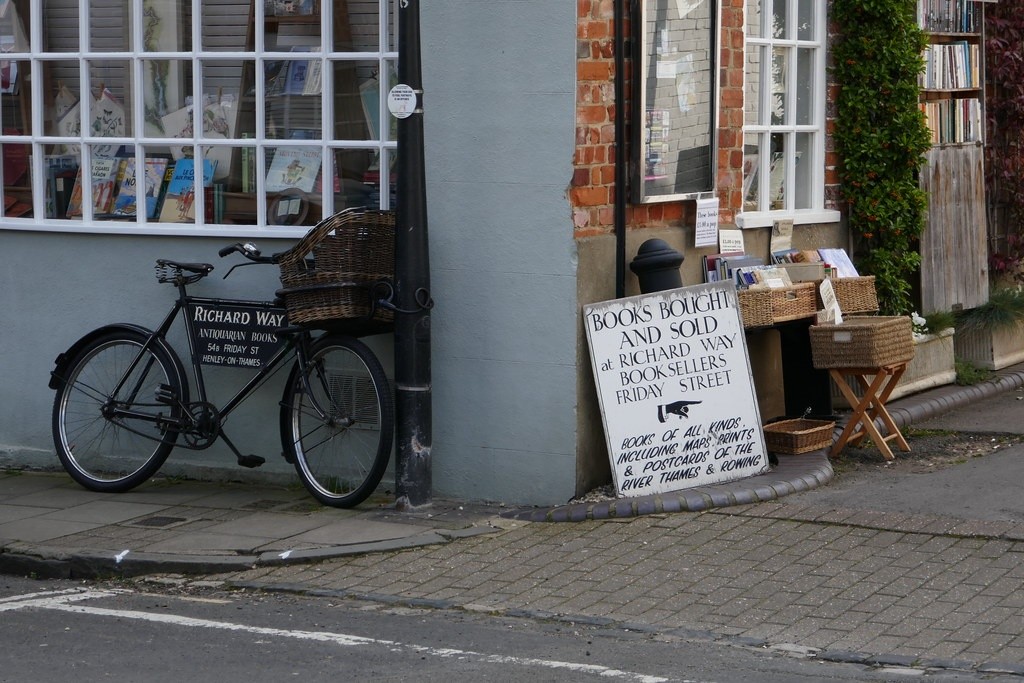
[917,0,983,143]
[0,0,395,225]
[702,248,860,291]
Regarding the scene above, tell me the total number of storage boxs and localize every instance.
[774,260,825,280]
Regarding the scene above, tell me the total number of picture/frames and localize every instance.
[358,68,398,156]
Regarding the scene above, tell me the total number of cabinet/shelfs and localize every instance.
[223,0,369,223]
[913,0,986,146]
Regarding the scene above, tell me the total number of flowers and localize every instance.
[910,311,957,343]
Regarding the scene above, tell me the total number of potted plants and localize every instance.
[956,288,1024,370]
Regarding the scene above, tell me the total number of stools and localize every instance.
[831,364,911,461]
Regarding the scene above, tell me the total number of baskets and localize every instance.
[762,418,836,455]
[793,275,880,313]
[737,282,818,329]
[809,315,915,368]
[275,208,395,336]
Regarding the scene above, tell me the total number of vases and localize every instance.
[831,327,957,408]
